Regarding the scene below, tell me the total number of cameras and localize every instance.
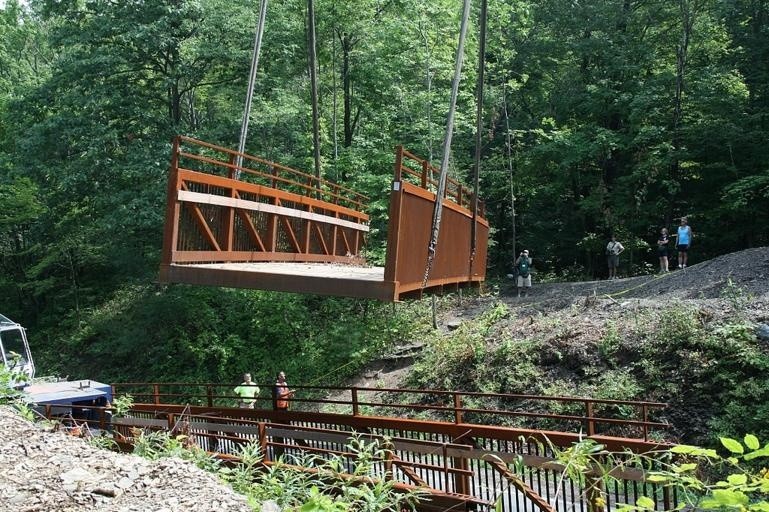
[521,252,526,257]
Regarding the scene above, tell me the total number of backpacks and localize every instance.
[519,256,530,279]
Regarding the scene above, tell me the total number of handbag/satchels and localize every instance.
[605,250,610,257]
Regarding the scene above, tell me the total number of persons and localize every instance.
[515,249,533,298]
[605,236,625,280]
[233,373,260,420]
[674,216,692,270]
[657,228,669,274]
[274,371,296,425]
